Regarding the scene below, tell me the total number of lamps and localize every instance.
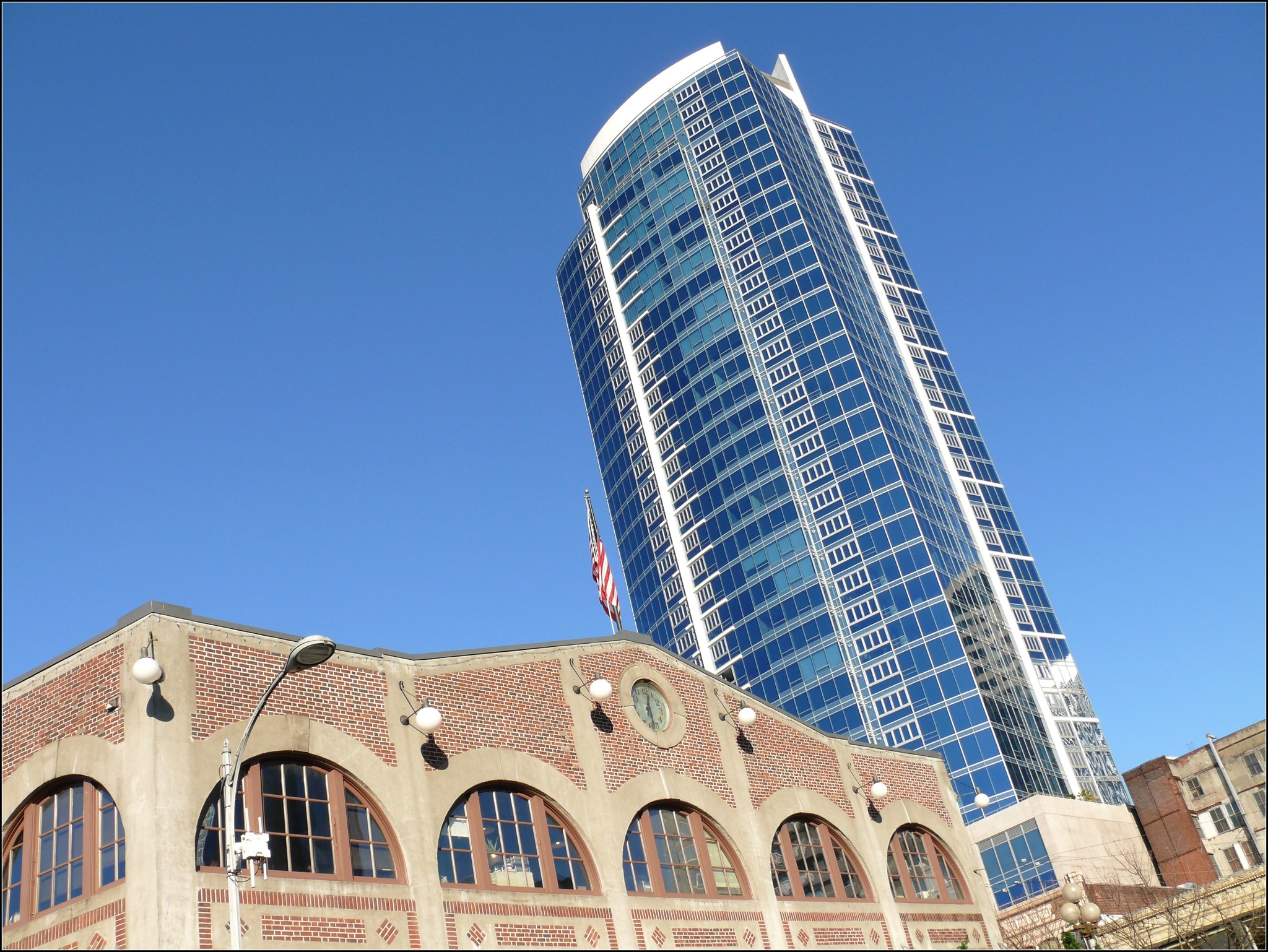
[713,688,756,728]
[846,764,889,802]
[568,657,613,704]
[131,631,166,687]
[397,679,442,734]
[944,771,991,809]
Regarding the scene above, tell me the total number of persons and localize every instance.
[306,864,318,873]
[208,859,220,867]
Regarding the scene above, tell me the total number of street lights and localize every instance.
[216,635,338,950]
[1059,882,1102,951]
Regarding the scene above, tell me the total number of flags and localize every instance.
[584,496,621,624]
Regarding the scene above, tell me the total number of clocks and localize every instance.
[630,677,673,734]
